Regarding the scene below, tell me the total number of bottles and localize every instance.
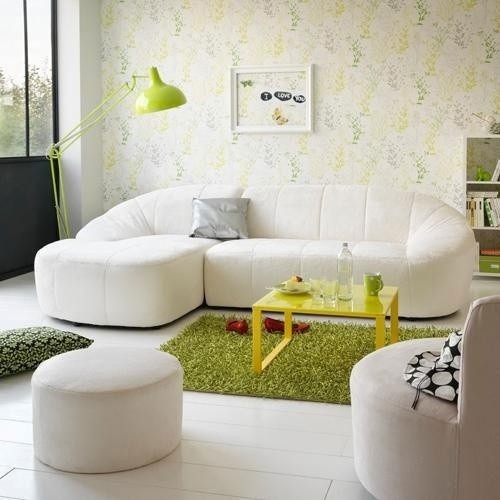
[336,242,354,302]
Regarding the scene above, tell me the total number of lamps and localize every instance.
[44,65,188,239]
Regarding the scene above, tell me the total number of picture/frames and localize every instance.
[227,63,315,133]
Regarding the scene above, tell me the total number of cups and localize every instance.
[363,274,384,297]
[310,278,326,300]
[320,279,338,305]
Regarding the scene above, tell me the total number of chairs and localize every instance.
[348,294,500,500]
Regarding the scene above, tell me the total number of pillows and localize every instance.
[0,326,94,379]
[190,197,251,240]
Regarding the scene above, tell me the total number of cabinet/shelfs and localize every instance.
[460,131,500,278]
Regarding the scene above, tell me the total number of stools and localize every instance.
[31,347,183,475]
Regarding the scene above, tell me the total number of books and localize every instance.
[467,190,500,229]
[491,160,500,181]
[481,249,500,257]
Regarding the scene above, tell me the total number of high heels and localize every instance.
[265,318,309,334]
[227,319,248,335]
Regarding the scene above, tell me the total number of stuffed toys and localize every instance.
[474,164,491,181]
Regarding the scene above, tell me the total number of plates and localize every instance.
[278,280,311,294]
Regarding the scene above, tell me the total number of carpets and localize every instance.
[159,313,464,406]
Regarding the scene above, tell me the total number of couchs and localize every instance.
[33,182,476,330]
